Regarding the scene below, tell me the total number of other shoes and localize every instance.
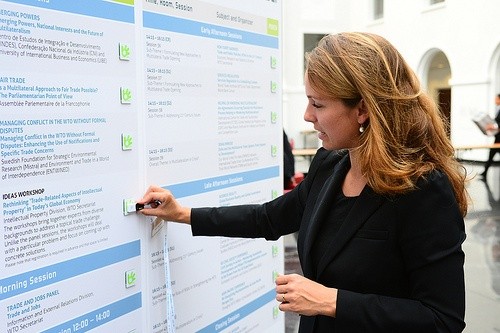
[481,172,486,177]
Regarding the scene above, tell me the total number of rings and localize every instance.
[281,294,287,302]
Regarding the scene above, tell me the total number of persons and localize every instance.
[136,32,471,333]
[480,93,500,175]
[283,128,295,190]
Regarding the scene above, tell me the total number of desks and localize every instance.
[292,148,317,163]
